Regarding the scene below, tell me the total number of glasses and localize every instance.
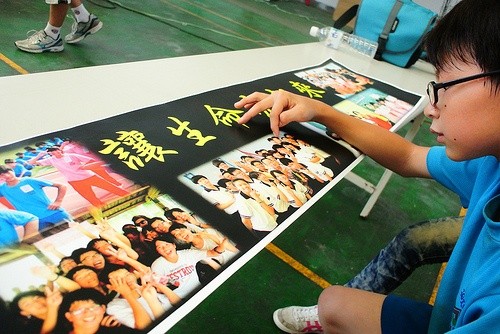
[136,219,146,226]
[81,253,101,263]
[69,304,100,316]
[427,73,497,105]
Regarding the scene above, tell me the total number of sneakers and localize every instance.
[67,14,104,44]
[272,305,323,334]
[14,29,65,53]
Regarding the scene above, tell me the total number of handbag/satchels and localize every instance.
[335,0,440,70]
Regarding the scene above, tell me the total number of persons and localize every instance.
[191,133,341,240]
[0,136,130,248]
[14,0,103,53]
[0,208,241,334]
[234,0,500,334]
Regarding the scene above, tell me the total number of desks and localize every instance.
[1,41,442,334]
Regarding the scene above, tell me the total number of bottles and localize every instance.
[310,26,378,62]
